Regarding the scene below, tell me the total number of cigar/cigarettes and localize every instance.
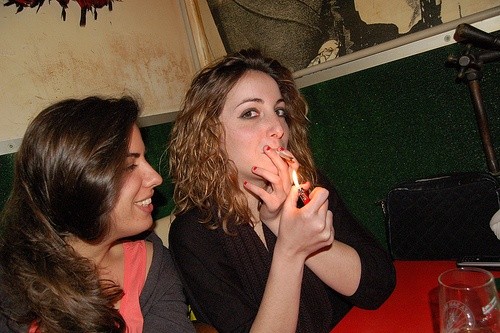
[274,149,295,163]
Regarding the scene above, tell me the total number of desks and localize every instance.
[324,256,500,332]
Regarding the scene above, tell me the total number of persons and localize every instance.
[166,45,398,333]
[0,94,196,333]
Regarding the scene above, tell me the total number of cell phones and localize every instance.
[456,254,500,270]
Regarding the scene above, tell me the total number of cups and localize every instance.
[438,267,500,333]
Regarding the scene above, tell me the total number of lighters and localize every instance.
[296,188,310,206]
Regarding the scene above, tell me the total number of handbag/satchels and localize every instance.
[374,169,500,260]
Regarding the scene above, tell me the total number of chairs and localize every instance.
[382,171,500,263]
